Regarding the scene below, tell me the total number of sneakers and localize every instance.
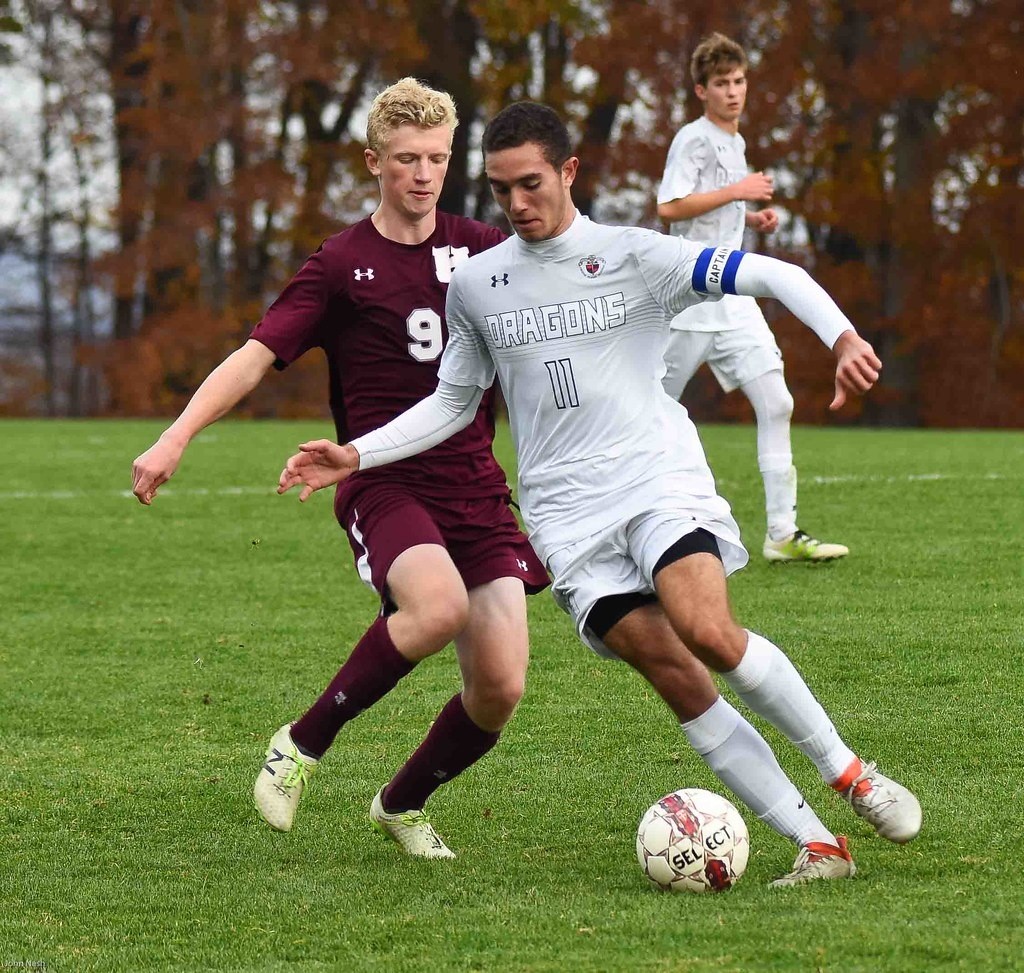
[762,529,849,564]
[842,758,923,843]
[369,782,457,858]
[254,721,319,832]
[769,836,856,890]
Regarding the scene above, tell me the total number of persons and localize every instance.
[657,33,851,562]
[275,99,924,888]
[129,77,552,858]
